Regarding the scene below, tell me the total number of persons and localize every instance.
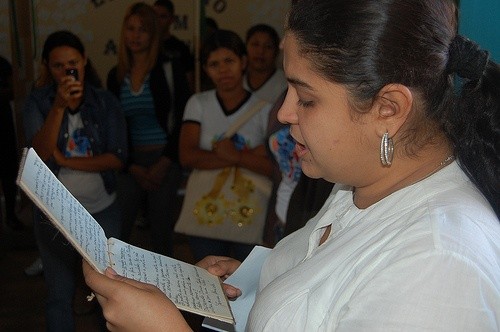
[180,30,282,263]
[152,0,194,137]
[0,56,26,231]
[240,24,288,102]
[82,0,500,332]
[105,3,178,256]
[24,30,128,332]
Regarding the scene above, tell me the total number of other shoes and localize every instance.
[24,256,45,277]
[5,209,24,232]
[74,292,97,314]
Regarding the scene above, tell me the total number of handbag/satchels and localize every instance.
[175,164,273,242]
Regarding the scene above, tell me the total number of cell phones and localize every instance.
[65,68,79,95]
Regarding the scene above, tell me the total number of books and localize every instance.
[16,147,235,325]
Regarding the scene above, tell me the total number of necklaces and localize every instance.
[406,154,454,187]
